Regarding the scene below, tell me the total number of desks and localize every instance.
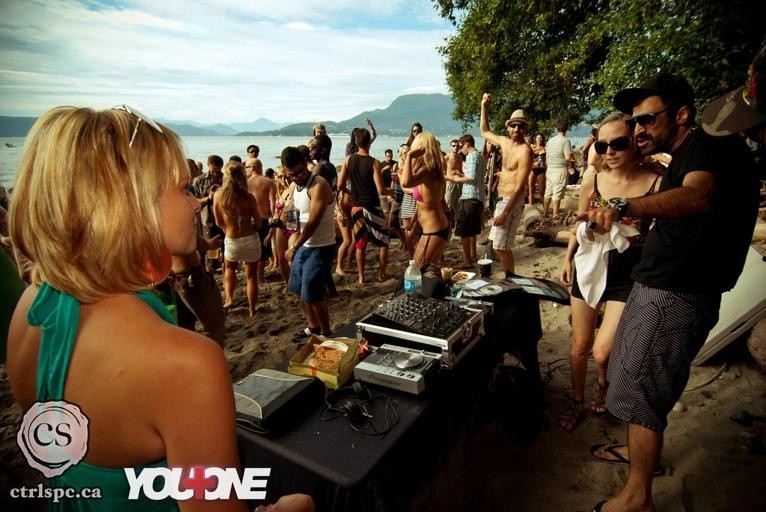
[235,275,543,512]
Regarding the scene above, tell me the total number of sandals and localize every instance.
[559,381,609,433]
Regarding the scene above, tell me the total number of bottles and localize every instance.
[404,259,422,295]
[571,145,576,161]
[286,196,298,231]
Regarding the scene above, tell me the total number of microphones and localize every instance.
[367,388,373,408]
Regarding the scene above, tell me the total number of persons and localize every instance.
[0,42,766,511]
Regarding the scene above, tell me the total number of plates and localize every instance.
[451,271,476,283]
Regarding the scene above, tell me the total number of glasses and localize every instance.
[459,143,465,149]
[287,165,307,178]
[625,108,671,131]
[452,145,459,147]
[111,105,163,148]
[509,123,525,128]
[595,136,634,154]
[245,166,254,169]
[248,150,258,154]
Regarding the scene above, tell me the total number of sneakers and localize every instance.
[298,327,329,332]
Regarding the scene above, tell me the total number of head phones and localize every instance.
[325,381,367,424]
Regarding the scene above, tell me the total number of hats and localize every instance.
[614,75,694,116]
[505,109,533,130]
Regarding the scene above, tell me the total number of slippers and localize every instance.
[590,444,663,476]
[591,501,608,512]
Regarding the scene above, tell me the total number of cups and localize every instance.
[441,267,453,280]
[478,259,492,278]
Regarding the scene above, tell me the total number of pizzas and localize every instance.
[310,342,346,374]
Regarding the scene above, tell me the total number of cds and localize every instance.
[409,356,427,369]
[394,352,424,370]
[480,285,503,295]
